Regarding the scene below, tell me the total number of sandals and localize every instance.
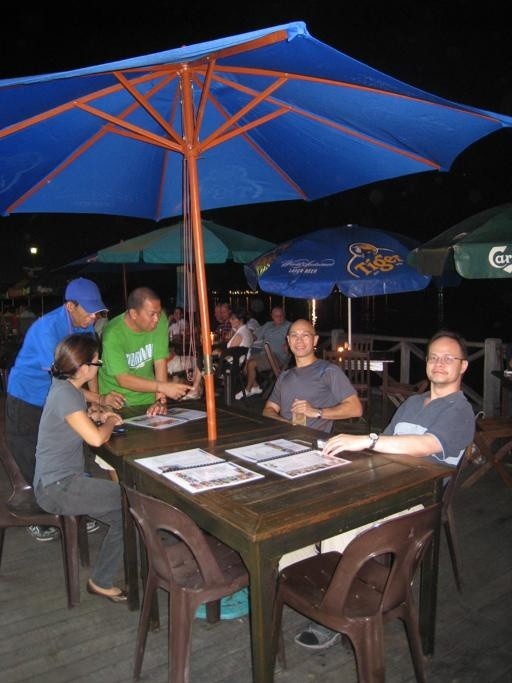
[181,392,201,401]
[294,621,345,649]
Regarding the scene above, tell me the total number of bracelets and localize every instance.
[156,379,161,393]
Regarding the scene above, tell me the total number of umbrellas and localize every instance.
[244,223,433,351]
[97,214,279,265]
[404,201,512,280]
[3,239,132,310]
[1,18,512,443]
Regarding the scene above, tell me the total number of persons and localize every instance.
[5,274,128,542]
[98,285,197,418]
[16,307,39,347]
[10,307,21,334]
[163,301,299,402]
[275,330,477,650]
[261,317,364,434]
[33,333,132,605]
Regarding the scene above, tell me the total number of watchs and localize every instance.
[316,407,323,419]
[369,431,379,451]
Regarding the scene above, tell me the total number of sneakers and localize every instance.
[235,384,263,400]
[26,522,60,540]
[88,521,100,533]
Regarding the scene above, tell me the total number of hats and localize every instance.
[65,278,110,314]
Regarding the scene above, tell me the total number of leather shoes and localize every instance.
[87,580,128,603]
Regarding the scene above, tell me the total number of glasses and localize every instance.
[426,351,464,365]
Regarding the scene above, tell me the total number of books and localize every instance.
[135,446,267,495]
[120,407,207,431]
[226,436,355,481]
